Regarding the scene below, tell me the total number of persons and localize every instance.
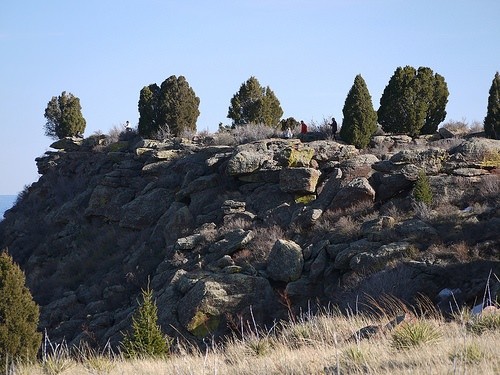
[123,120,131,131]
[301,120,308,141]
[328,117,338,141]
[284,125,291,138]
